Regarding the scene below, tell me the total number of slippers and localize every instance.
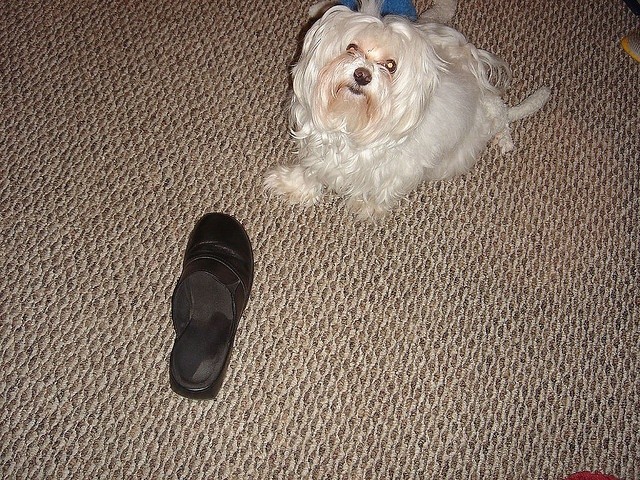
[169,212,254,401]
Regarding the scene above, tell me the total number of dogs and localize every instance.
[255,0,553,221]
